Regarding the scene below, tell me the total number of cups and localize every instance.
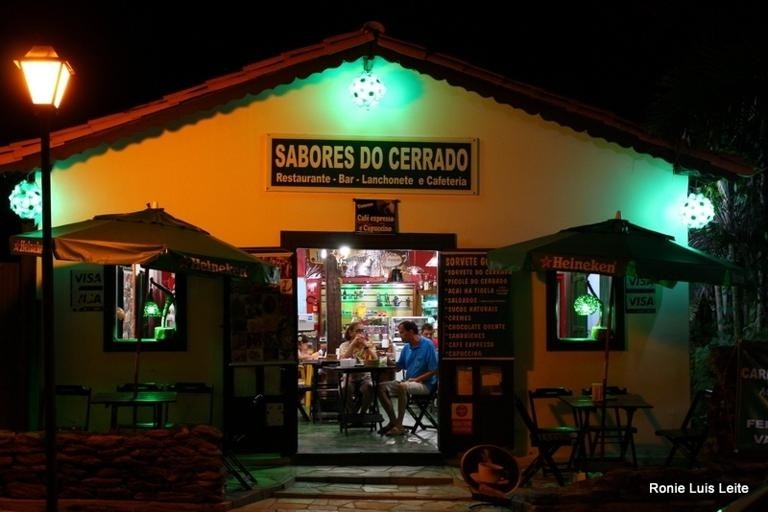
[377,355,388,367]
[592,376,605,404]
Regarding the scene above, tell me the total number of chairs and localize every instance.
[37,382,265,492]
[297,383,312,422]
[653,387,715,472]
[312,359,342,425]
[399,378,438,435]
[516,385,638,487]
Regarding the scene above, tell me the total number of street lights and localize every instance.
[10,40,83,511]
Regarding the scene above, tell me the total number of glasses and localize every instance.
[352,329,365,334]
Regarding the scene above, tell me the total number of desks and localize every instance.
[557,392,655,471]
[322,364,400,436]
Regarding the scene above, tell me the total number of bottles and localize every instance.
[376,291,411,307]
[340,286,367,299]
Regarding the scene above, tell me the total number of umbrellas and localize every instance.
[9,202,280,430]
[483,210,749,468]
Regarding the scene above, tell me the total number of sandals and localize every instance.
[378,423,405,435]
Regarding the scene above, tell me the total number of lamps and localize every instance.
[679,176,715,230]
[8,167,42,219]
[142,277,179,342]
[347,56,386,108]
[573,280,615,343]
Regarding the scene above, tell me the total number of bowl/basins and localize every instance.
[339,358,357,367]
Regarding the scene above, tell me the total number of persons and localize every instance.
[299,319,438,437]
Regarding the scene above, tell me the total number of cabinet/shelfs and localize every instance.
[319,283,418,343]
